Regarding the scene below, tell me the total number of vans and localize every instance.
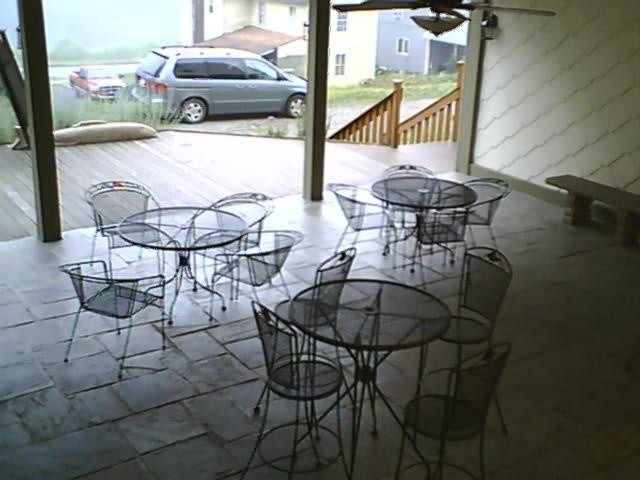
[124,42,307,127]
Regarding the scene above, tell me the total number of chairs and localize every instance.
[57,260,167,381]
[84,178,163,274]
[208,230,304,321]
[190,192,275,276]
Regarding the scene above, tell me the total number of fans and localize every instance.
[332,0,556,37]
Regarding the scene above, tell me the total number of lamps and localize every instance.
[478,14,500,40]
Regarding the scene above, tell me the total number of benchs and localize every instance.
[545,174,639,245]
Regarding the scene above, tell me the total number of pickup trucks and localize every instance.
[65,63,129,104]
